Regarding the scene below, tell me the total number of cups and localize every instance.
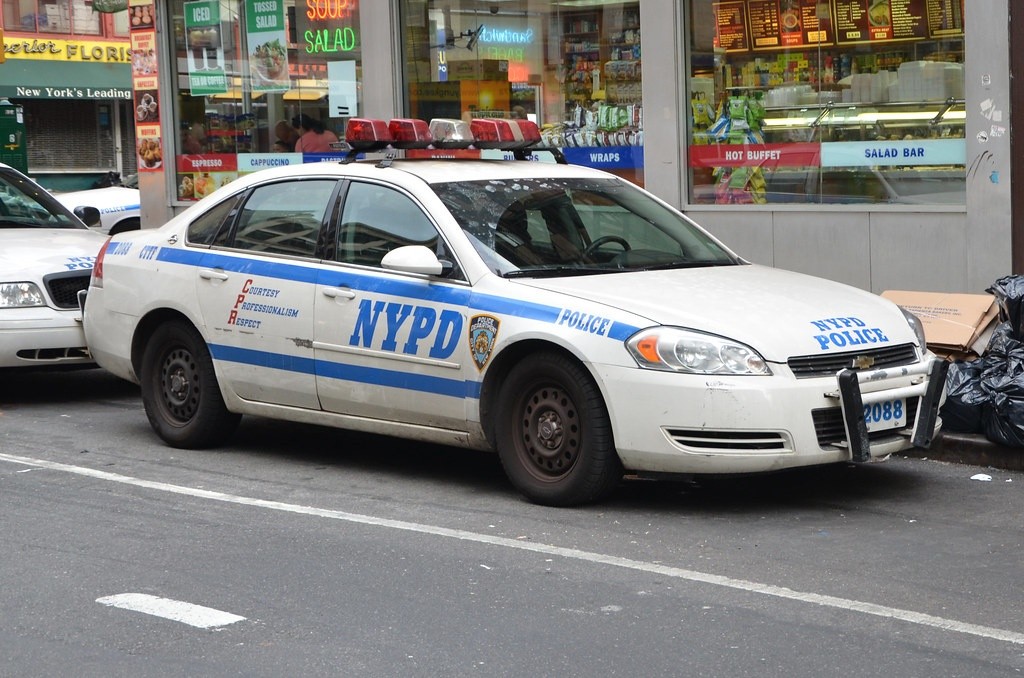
[206,46,218,70]
[149,102,157,113]
[137,105,147,119]
[192,45,205,70]
[143,94,151,105]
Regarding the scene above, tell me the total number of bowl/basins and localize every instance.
[255,56,285,80]
[784,14,798,28]
[759,61,964,109]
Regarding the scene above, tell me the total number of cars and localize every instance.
[82,115,949,509]
[46,172,141,240]
[1,158,117,380]
[203,110,258,149]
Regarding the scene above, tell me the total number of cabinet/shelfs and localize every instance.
[761,100,966,175]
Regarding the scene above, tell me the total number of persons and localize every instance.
[272,114,341,153]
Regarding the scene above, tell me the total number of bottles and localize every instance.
[721,48,908,99]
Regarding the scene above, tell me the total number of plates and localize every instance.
[138,108,149,122]
[868,0,891,27]
[139,158,162,170]
[147,110,158,118]
[141,95,154,108]
